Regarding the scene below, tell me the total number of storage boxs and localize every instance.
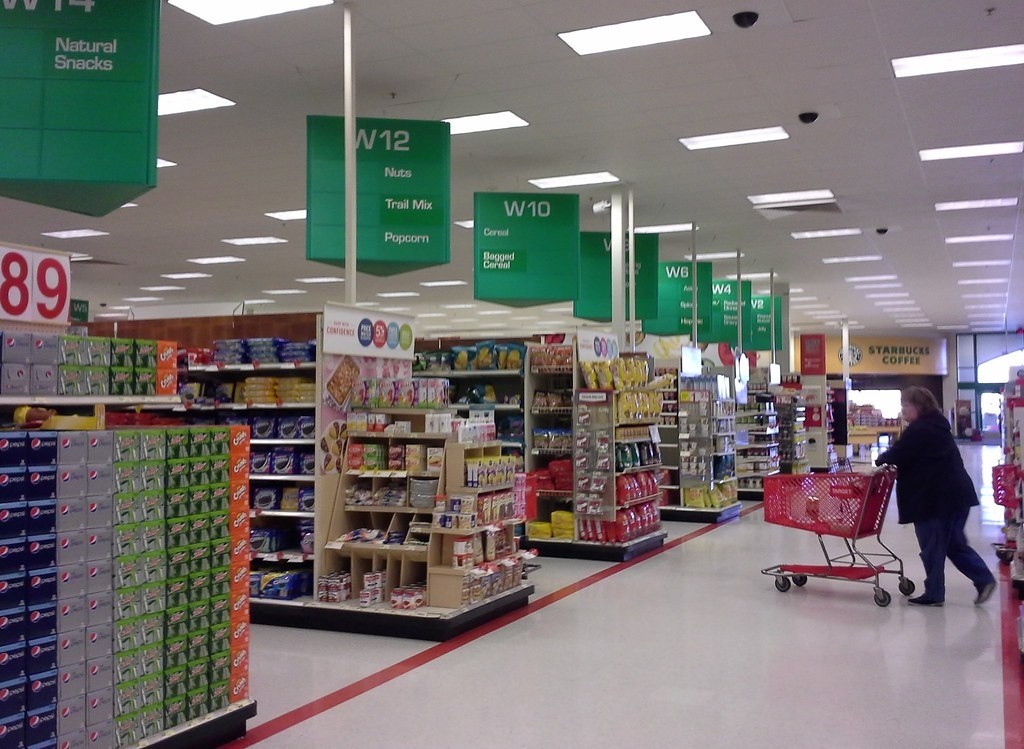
[317,374,528,608]
[0,328,317,749]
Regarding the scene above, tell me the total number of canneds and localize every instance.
[393,581,427,609]
[360,569,387,607]
[318,572,351,602]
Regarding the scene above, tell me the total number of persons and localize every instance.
[875,386,997,605]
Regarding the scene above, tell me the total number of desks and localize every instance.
[847,426,900,444]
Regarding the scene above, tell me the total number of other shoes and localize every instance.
[907,593,944,606]
[974,580,997,604]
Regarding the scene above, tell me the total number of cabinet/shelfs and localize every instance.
[676,398,741,523]
[660,388,684,521]
[315,408,450,643]
[428,438,535,642]
[577,386,667,563]
[528,362,576,557]
[415,369,524,446]
[186,361,315,630]
[735,410,782,500]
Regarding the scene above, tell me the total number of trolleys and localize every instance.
[991,463,1024,564]
[760,457,917,607]
